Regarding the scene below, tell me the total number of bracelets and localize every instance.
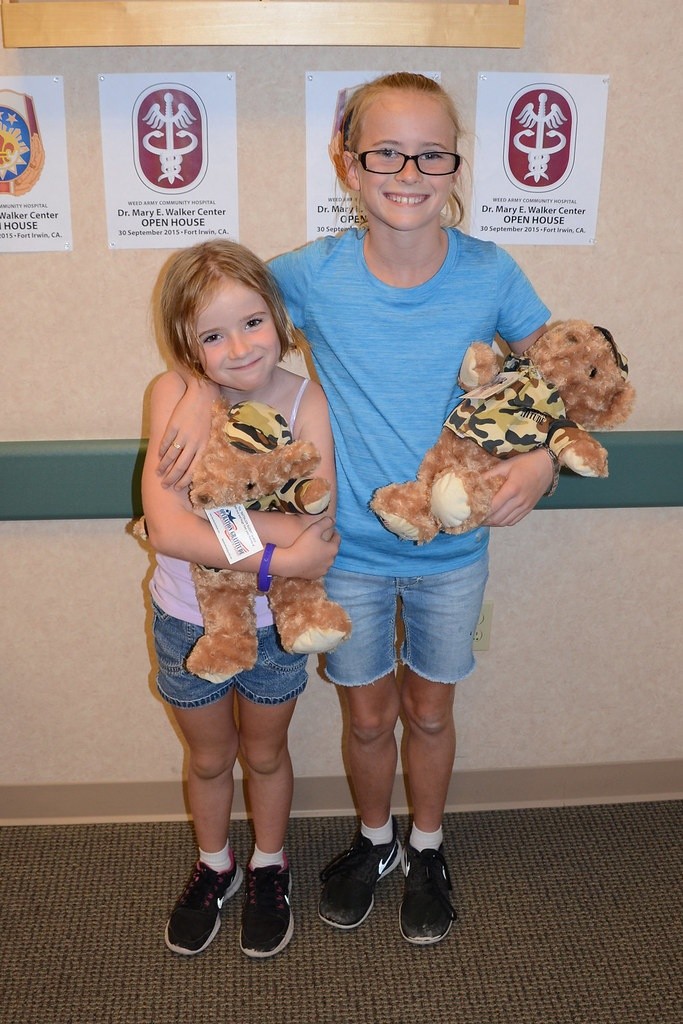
[252,541,279,593]
[536,441,560,498]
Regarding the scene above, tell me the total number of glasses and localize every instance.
[351,148,462,176]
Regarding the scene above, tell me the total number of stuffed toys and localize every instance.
[369,308,633,545]
[133,394,354,686]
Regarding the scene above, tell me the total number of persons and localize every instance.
[133,240,342,962]
[155,65,562,947]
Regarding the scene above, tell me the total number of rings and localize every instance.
[172,440,184,450]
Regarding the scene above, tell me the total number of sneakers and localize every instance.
[316,816,454,944]
[239,849,294,958]
[164,860,243,955]
